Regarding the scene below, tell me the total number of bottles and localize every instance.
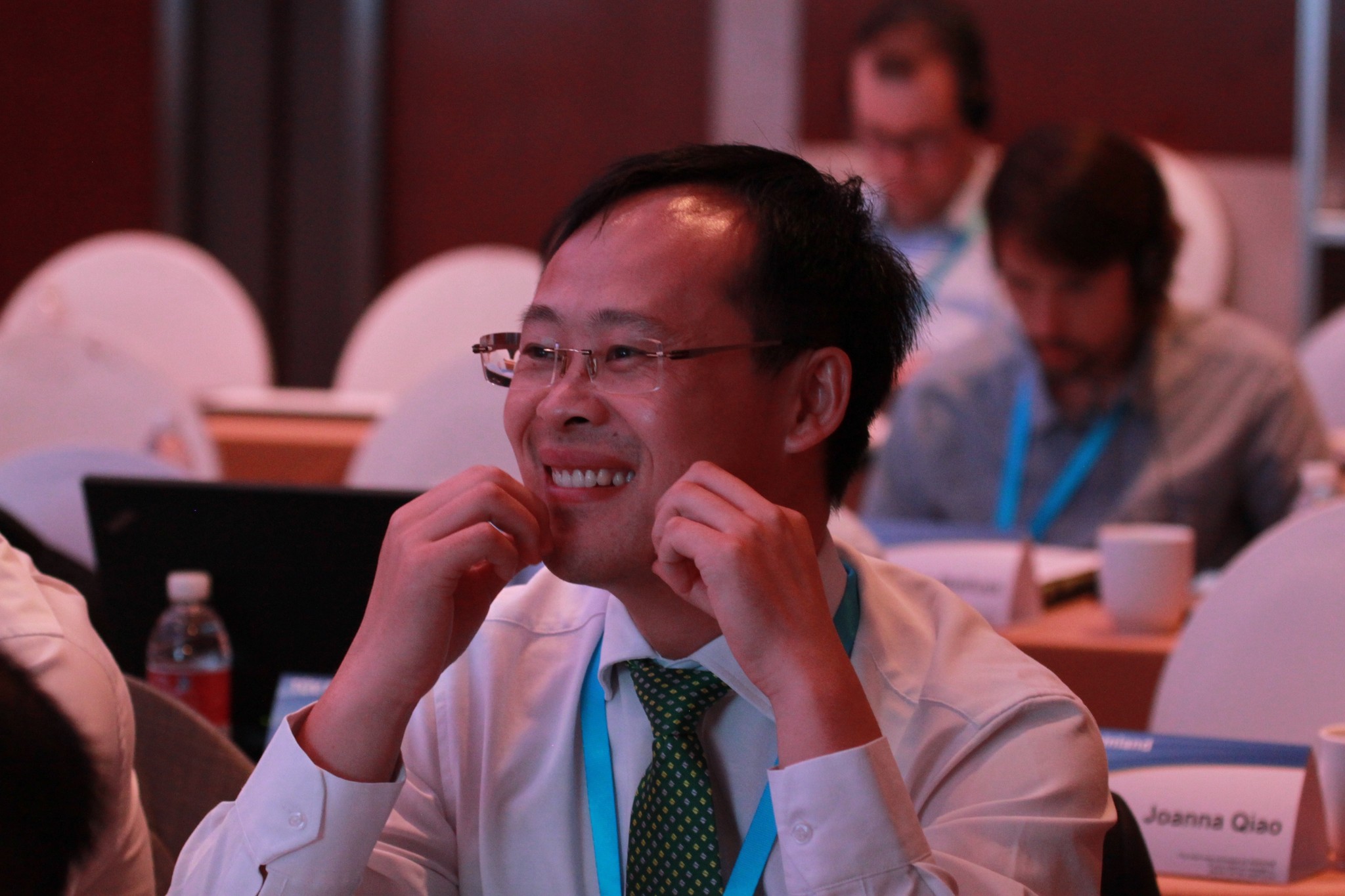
[1293,459,1342,514]
[145,571,231,739]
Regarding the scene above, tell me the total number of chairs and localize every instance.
[0,236,566,896]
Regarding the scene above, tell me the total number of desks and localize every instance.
[216,391,1345,896]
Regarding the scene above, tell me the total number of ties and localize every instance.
[625,659,730,896]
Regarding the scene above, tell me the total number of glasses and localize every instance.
[474,332,792,395]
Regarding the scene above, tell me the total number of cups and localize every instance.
[1097,518,1203,634]
[1319,724,1345,868]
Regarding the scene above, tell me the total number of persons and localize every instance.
[770,0,1035,436]
[840,121,1323,587]
[147,131,1124,894]
[0,523,162,896]
